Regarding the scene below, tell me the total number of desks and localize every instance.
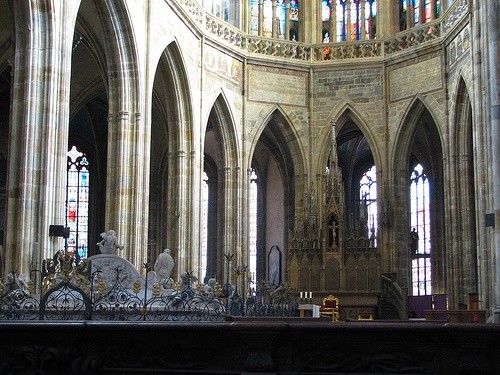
[298,304,321,318]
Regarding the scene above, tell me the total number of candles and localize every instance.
[305,292,307,298]
[300,292,302,298]
[310,292,312,298]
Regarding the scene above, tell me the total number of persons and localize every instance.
[154,248,174,283]
[97,230,124,250]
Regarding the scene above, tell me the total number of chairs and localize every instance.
[321,295,339,322]
